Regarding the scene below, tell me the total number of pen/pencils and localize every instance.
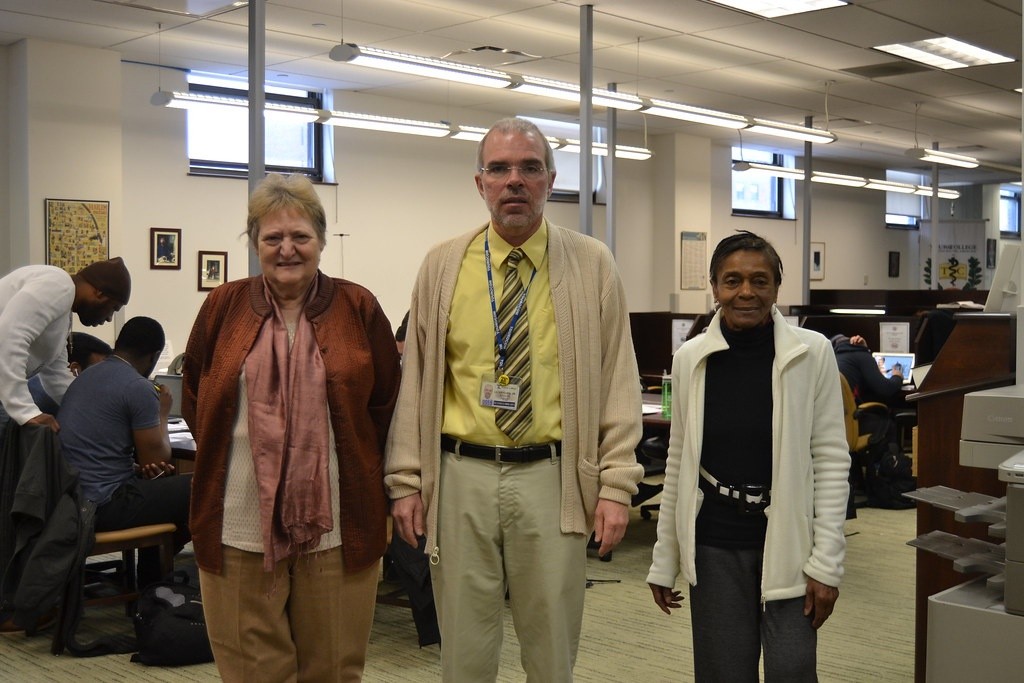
[153,384,161,393]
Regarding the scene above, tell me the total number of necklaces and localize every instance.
[114,354,132,366]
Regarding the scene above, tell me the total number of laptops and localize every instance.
[154,374,185,423]
[872,352,915,385]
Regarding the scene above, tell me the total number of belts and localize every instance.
[441,434,562,465]
[699,463,772,510]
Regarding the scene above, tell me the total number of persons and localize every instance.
[67,328,111,378]
[58,316,197,596]
[179,172,401,683]
[0,256,130,636]
[395,326,406,359]
[646,228,852,683]
[831,332,905,495]
[382,117,646,683]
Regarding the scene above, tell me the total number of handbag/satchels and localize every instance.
[131,570,215,666]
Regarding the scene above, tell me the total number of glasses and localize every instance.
[480,165,548,180]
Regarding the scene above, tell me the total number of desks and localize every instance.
[642,392,673,423]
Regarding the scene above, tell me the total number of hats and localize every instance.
[78,257,131,305]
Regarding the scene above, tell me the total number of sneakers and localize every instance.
[0,610,60,635]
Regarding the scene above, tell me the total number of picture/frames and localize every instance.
[887,250,901,279]
[198,250,228,291]
[150,227,182,270]
[810,240,825,281]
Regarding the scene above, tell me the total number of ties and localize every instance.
[494,248,533,449]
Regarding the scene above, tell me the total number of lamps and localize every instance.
[164,43,979,198]
[151,22,175,108]
[329,1,361,63]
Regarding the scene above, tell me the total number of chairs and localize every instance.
[10,420,178,653]
[838,370,891,520]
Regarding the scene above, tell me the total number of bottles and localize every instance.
[662,377,671,419]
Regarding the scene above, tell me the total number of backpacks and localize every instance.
[869,447,917,509]
